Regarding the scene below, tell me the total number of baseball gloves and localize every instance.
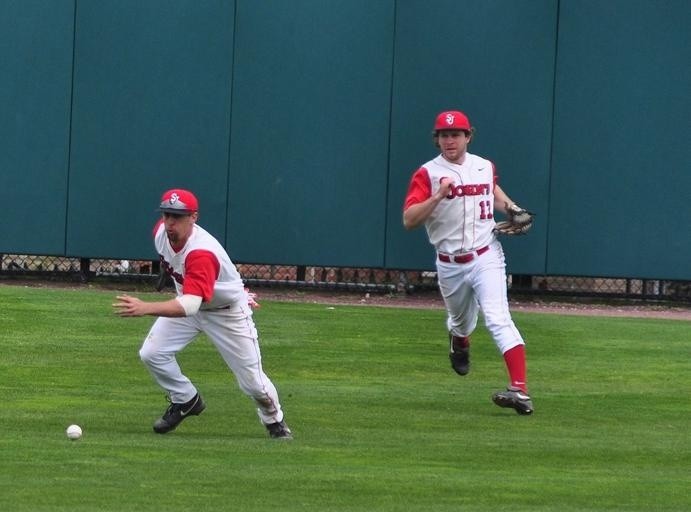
[494,202,536,235]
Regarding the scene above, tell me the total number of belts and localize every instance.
[439,246,489,263]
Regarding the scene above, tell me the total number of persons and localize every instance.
[111,189,292,439]
[403,111,534,414]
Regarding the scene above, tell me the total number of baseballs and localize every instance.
[67,425,82,440]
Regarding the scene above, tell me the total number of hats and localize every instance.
[160,190,198,215]
[436,112,470,131]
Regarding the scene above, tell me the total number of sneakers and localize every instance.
[493,387,533,415]
[153,392,205,433]
[449,335,469,376]
[266,420,291,438]
